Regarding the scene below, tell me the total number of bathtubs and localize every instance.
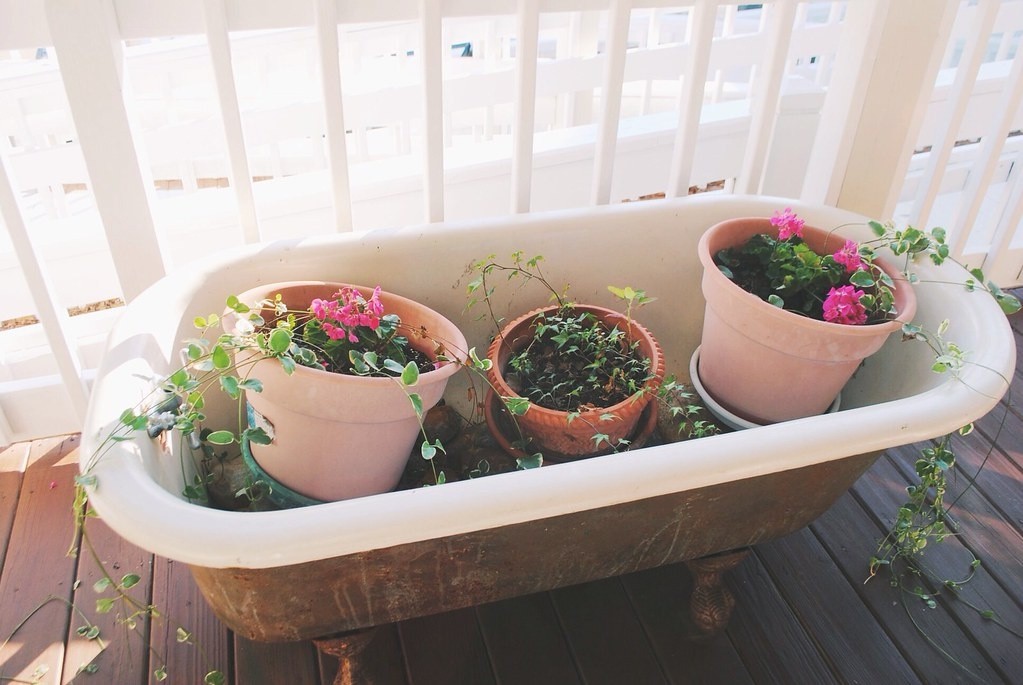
[76,195,1018,646]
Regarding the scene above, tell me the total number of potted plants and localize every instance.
[485,299,663,469]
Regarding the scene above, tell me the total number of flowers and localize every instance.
[765,209,873,332]
[309,279,393,349]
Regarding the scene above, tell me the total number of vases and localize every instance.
[219,279,471,510]
[687,217,918,432]
